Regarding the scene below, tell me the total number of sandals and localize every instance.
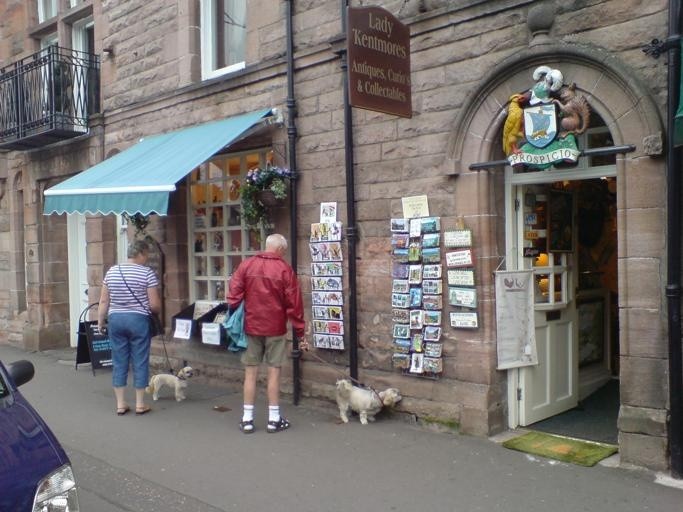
[238,416,255,433]
[267,417,291,433]
[136,405,150,414]
[117,406,129,416]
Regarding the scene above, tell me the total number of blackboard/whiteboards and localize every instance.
[85,319,113,369]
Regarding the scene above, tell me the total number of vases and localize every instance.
[254,189,286,223]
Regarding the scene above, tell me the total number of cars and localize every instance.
[0,359,79,511]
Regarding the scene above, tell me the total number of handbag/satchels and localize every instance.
[148,312,165,337]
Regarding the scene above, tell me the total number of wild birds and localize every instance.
[502,94,529,157]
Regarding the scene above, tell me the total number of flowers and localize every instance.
[239,163,298,242]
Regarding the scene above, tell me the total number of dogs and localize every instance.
[334,377,402,426]
[144,366,194,402]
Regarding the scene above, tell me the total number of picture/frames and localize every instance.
[521,102,558,149]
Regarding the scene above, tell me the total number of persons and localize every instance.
[226,234,308,433]
[98,239,160,414]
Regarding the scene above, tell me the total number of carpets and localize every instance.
[502,430,619,467]
